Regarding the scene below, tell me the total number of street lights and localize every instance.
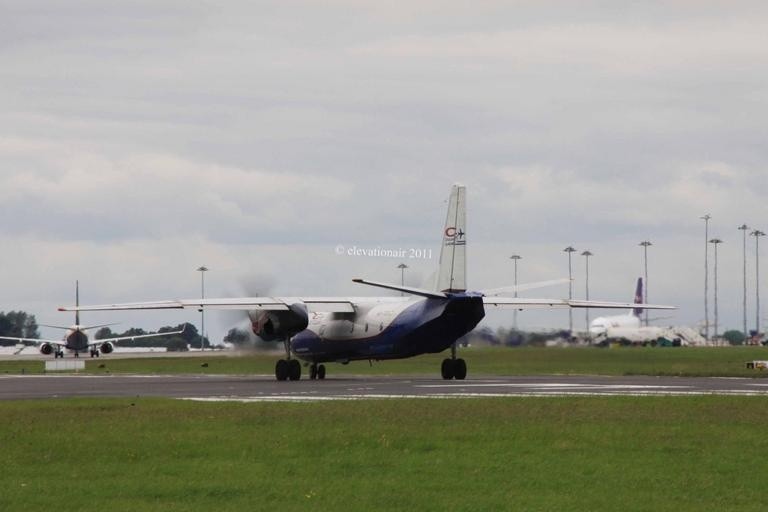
[578,248,591,337]
[638,241,654,326]
[509,254,521,299]
[708,239,729,346]
[749,229,764,334]
[562,246,576,340]
[699,215,714,337]
[398,262,407,299]
[736,225,751,337]
[195,266,207,353]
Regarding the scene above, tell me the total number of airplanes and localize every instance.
[584,277,677,336]
[53,182,681,384]
[0,280,191,360]
